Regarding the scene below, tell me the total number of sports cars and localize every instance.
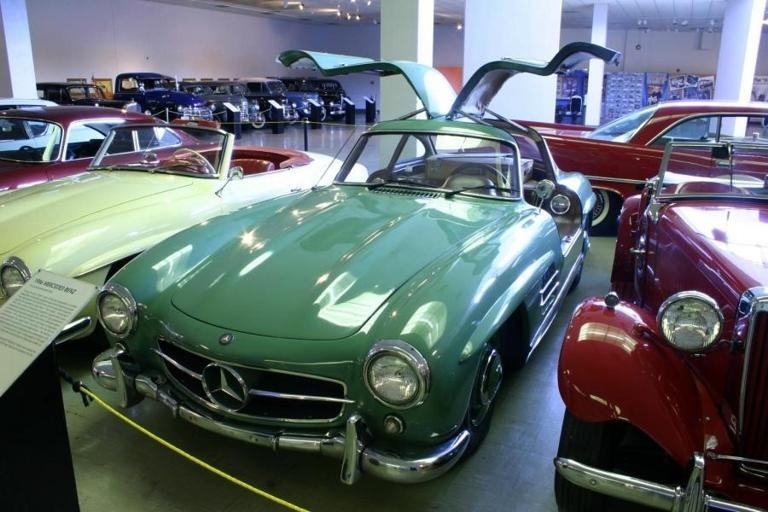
[0,118,371,354]
[83,41,600,489]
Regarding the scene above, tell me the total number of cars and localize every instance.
[548,139,767,512]
[0,106,240,200]
[460,91,768,238]
[0,98,120,154]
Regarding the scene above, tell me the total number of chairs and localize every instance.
[218,157,276,179]
[674,179,752,201]
